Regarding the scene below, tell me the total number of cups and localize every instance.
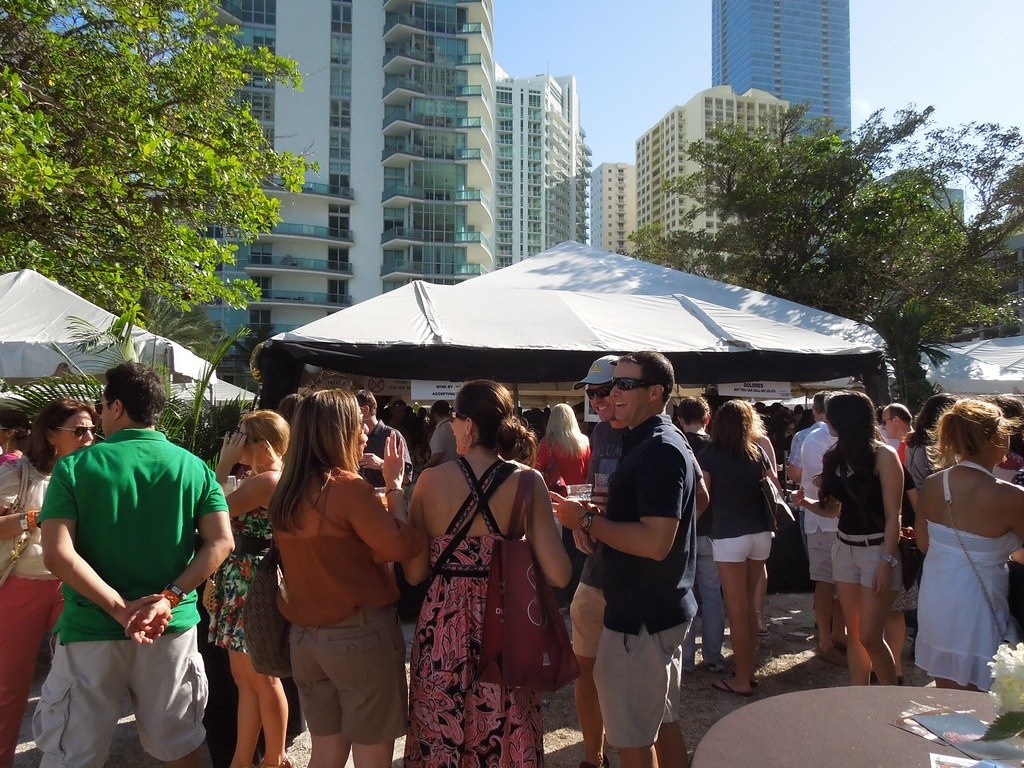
[594,473,610,508]
[566,485,592,510]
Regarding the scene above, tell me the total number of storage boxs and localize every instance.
[301,366,585,412]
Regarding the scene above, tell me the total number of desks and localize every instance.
[689,686,1024,768]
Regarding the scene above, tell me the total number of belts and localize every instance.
[835,531,885,547]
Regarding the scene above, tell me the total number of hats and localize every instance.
[573,355,621,390]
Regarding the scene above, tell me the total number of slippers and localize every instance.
[710,668,757,697]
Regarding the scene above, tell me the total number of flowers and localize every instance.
[973,643,1024,742]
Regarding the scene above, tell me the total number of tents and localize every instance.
[268,239,891,412]
[799,334,1024,397]
[0,269,217,384]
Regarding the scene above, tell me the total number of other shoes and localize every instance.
[756,610,769,636]
[815,643,848,664]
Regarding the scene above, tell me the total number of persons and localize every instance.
[905,392,958,489]
[0,410,29,455]
[202,409,290,768]
[39,358,235,768]
[0,396,96,768]
[0,351,1024,768]
[270,350,1024,768]
[276,394,304,422]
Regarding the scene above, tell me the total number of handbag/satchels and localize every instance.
[755,442,796,533]
[0,455,30,586]
[543,443,568,499]
[1002,614,1021,651]
[479,466,580,693]
[241,546,292,680]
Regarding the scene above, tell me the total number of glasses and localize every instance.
[585,385,612,400]
[240,433,265,444]
[55,425,97,437]
[881,417,893,425]
[609,376,669,391]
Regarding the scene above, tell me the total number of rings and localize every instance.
[229,441,233,443]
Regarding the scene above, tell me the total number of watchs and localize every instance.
[879,552,898,567]
[579,511,595,535]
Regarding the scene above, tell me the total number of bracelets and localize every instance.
[385,487,404,498]
[27,511,37,529]
[165,583,186,600]
[161,590,180,608]
[20,513,29,531]
